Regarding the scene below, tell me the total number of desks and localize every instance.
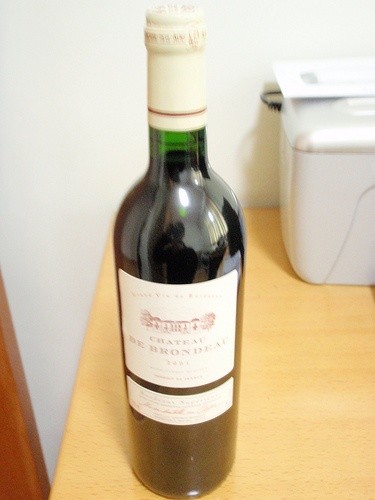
[46,206,375,500]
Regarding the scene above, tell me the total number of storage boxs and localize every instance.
[276,93,375,286]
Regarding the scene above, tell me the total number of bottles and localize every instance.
[114,7,246,500]
